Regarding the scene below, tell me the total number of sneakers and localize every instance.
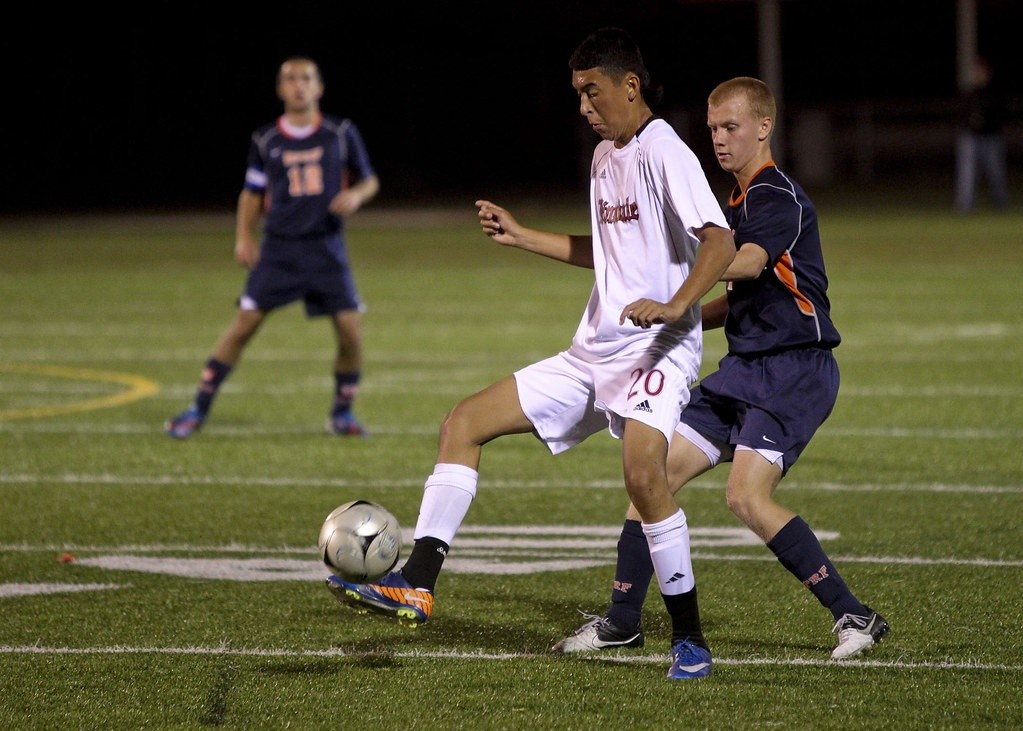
[830,605,890,659]
[552,608,644,654]
[667,636,713,680]
[330,411,365,436]
[327,571,434,629]
[165,400,204,439]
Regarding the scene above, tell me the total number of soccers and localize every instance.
[318,498,403,585]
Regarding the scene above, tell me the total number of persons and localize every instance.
[164,56,378,441]
[326,27,737,679]
[548,79,890,661]
